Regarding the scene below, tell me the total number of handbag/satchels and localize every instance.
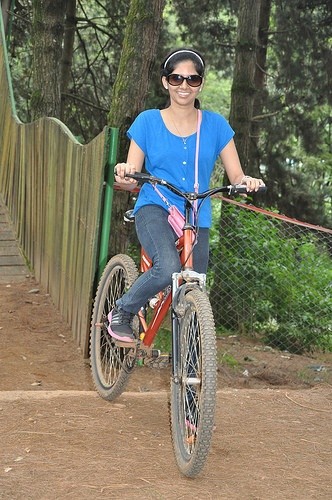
[167,205,197,245]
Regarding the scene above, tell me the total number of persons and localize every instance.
[106,47,251,444]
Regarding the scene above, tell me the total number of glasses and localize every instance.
[167,73,203,87]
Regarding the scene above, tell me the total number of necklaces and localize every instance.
[168,108,197,144]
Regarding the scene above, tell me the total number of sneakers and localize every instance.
[185,410,217,433]
[107,306,135,343]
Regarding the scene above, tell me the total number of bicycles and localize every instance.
[89,172,268,478]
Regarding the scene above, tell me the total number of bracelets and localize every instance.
[240,176,250,183]
